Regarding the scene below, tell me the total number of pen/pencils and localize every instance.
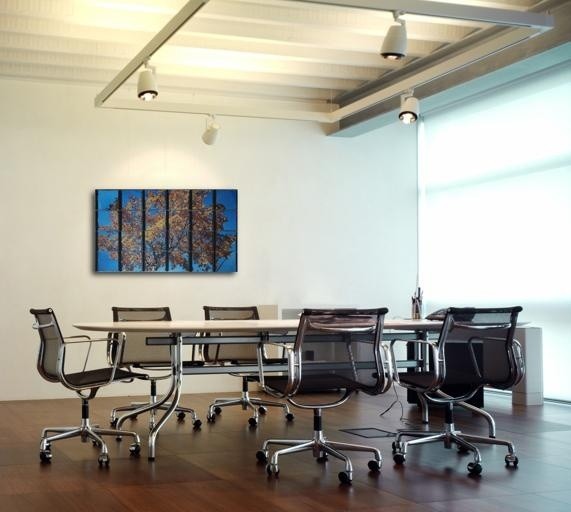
[412,287,423,319]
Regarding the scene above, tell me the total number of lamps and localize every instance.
[94,1,555,123]
